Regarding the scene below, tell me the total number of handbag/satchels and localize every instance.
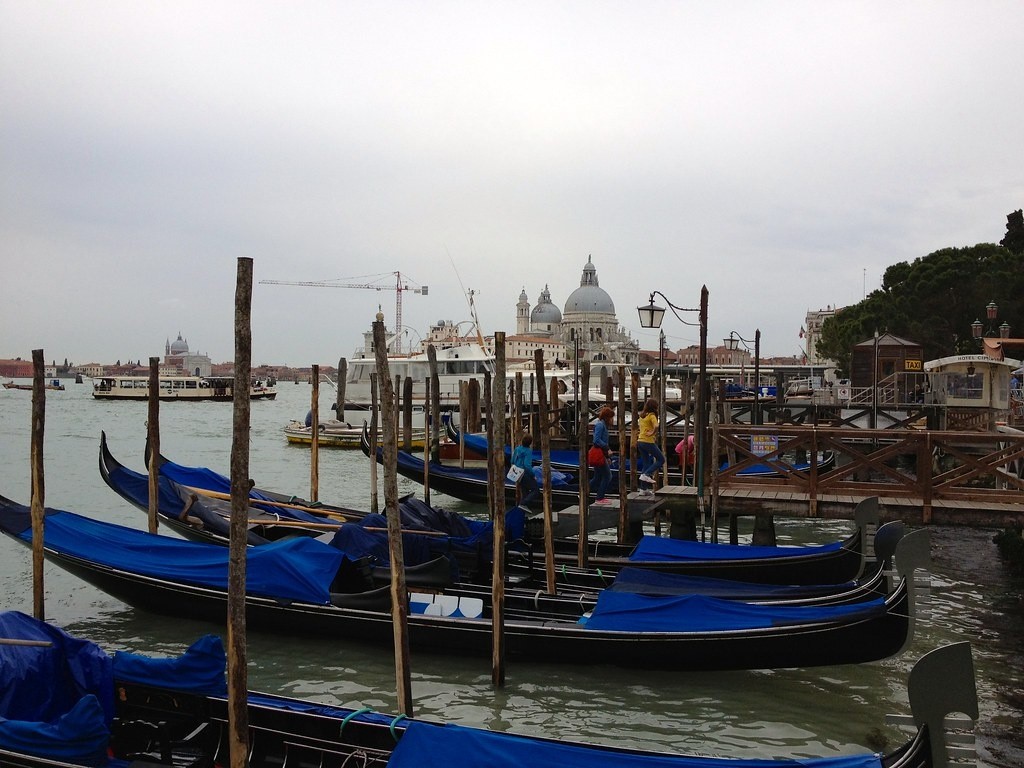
[587,445,605,468]
[506,464,525,484]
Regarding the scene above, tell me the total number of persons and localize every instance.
[578,407,616,505]
[201,378,230,395]
[675,435,694,474]
[305,409,311,427]
[506,433,538,514]
[908,385,924,404]
[251,375,273,387]
[1009,372,1019,400]
[637,397,666,496]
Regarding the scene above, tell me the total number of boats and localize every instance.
[328,258,695,414]
[4,381,67,390]
[93,330,279,402]
[0,598,928,768]
[1,378,929,665]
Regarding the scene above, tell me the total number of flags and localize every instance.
[799,326,806,339]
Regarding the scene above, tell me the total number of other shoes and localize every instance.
[595,499,613,505]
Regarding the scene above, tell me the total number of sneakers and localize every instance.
[518,504,532,514]
[640,489,652,496]
[639,474,656,484]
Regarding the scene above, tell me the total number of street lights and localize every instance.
[637,283,709,521]
[723,329,761,425]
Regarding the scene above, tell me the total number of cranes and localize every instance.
[252,271,430,358]
